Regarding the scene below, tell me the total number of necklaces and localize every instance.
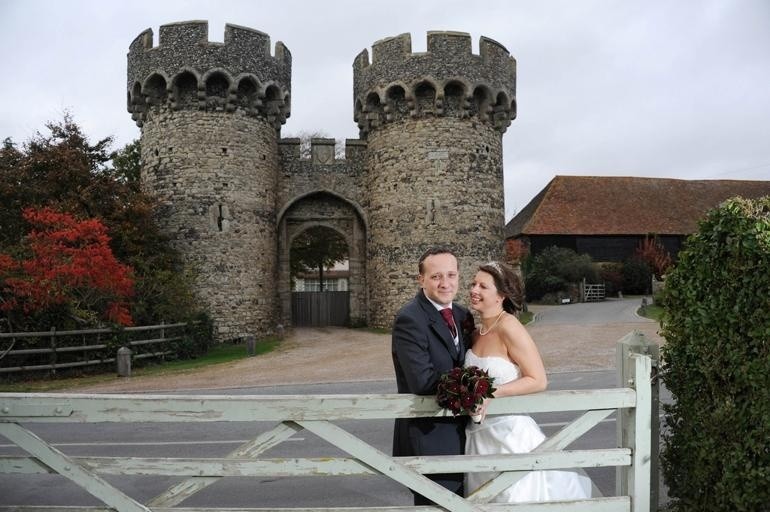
[478,310,507,337]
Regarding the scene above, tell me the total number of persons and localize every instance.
[391,247,477,507]
[460,260,603,501]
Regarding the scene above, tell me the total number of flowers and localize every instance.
[436,365,497,424]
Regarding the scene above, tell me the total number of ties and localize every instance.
[439,308,457,339]
